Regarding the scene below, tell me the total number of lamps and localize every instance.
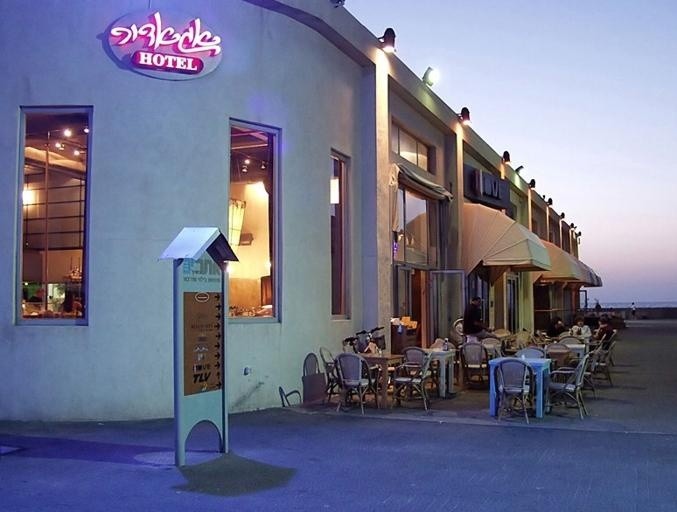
[377,26,553,207]
[228,197,246,257]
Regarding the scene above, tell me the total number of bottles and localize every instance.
[441,338,449,352]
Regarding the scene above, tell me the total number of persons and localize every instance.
[631,302,637,319]
[553,316,569,336]
[571,317,592,339]
[60,290,82,313]
[596,302,601,310]
[29,288,43,303]
[589,318,614,359]
[463,296,496,339]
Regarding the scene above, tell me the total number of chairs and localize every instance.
[320,322,619,423]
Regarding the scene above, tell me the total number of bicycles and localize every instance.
[344,327,390,386]
